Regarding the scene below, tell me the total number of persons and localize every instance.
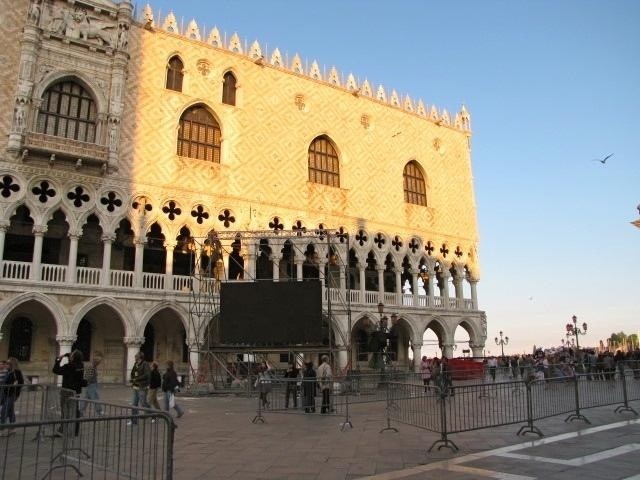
[316,354,334,414]
[0,360,8,411]
[441,355,455,397]
[432,357,441,393]
[126,350,158,426]
[300,359,320,413]
[161,358,185,420]
[485,346,640,383]
[283,360,299,409]
[147,362,162,424]
[50,347,84,437]
[420,356,432,393]
[0,354,24,437]
[81,354,110,419]
[257,360,274,409]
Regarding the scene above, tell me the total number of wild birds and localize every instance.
[590,153,614,165]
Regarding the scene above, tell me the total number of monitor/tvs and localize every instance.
[219,281,323,347]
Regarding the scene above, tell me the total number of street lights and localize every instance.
[566,315,588,372]
[494,329,509,360]
[377,302,398,369]
[562,333,574,349]
